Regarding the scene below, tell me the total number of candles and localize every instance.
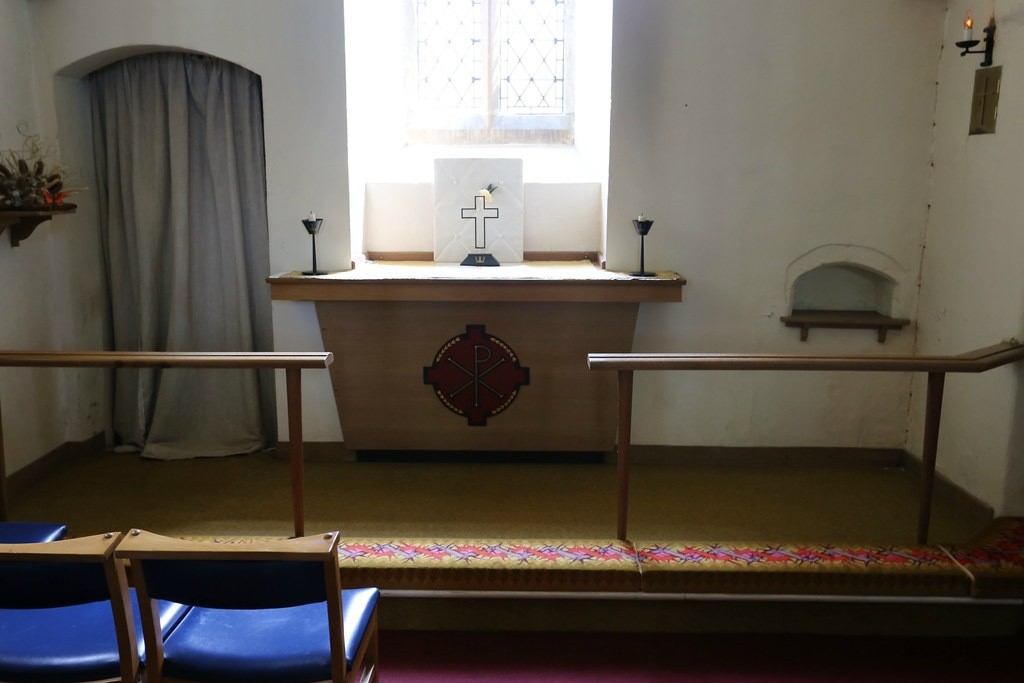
[638,212,646,221]
[308,211,316,221]
[963,17,974,40]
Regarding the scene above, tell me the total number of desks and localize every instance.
[265,259,687,452]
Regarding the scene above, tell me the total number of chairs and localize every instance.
[0,522,385,683]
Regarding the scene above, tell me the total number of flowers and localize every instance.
[0,122,90,211]
[479,183,498,204]
